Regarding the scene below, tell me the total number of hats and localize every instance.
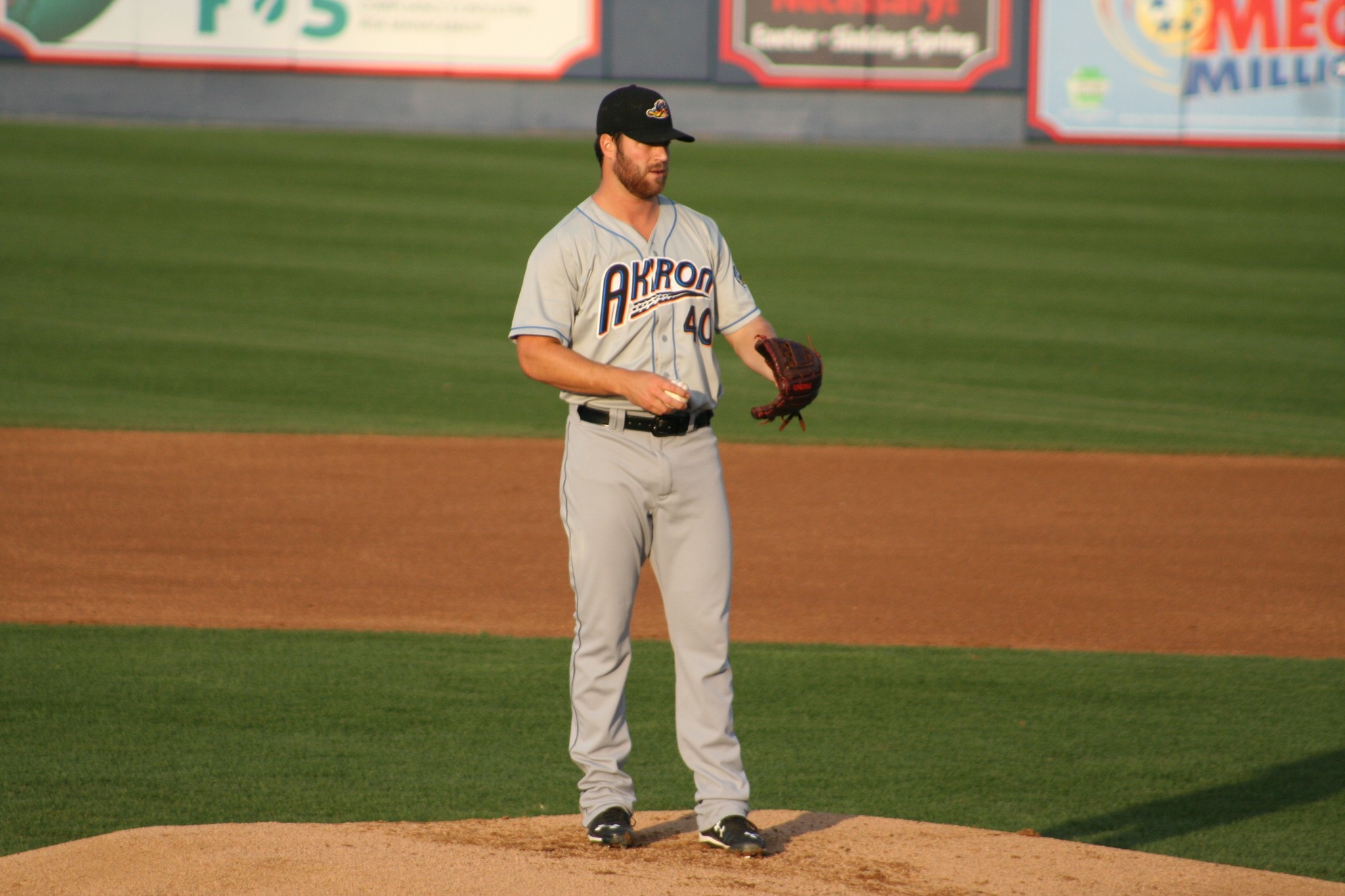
[595,84,695,144]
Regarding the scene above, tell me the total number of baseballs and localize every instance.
[663,379,690,409]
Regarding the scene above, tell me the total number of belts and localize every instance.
[577,404,714,437]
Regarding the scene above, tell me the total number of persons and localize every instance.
[508,80,786,853]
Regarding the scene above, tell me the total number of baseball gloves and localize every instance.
[750,334,823,421]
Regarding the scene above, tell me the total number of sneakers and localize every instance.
[588,805,637,847]
[699,814,766,856]
